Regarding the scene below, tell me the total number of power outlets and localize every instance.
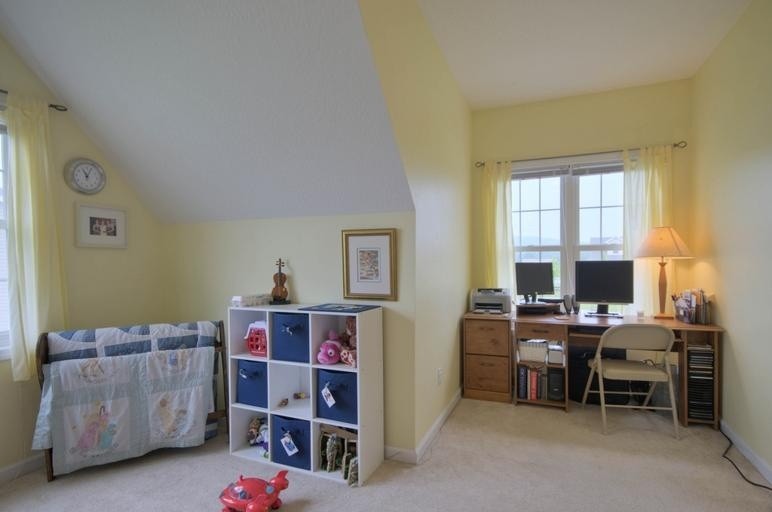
[438,367,445,385]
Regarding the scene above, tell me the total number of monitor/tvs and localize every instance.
[516,262,554,305]
[575,261,633,318]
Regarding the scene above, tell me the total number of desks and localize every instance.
[511,312,726,430]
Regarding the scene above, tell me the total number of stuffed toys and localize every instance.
[318,317,357,367]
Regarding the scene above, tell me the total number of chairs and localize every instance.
[582,323,680,438]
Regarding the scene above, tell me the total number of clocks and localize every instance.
[63,157,107,195]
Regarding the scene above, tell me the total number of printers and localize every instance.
[470,287,511,314]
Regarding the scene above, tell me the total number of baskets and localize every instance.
[518,340,547,363]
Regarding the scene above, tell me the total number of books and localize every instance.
[672,288,712,325]
[518,366,541,401]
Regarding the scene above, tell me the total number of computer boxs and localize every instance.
[516,303,560,315]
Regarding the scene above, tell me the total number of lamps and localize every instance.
[635,226,697,319]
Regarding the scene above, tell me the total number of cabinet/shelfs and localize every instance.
[227,303,386,488]
[463,311,512,402]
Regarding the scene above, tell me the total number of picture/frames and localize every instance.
[341,229,397,300]
[73,204,128,249]
